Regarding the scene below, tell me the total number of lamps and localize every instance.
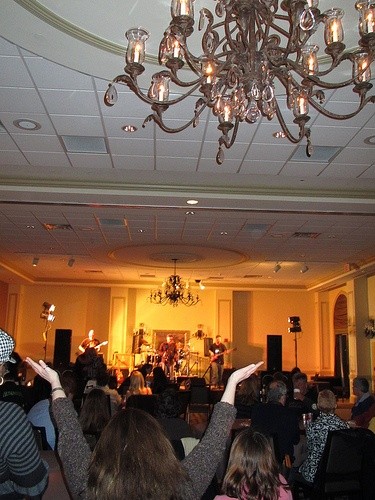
[273,261,281,272]
[147,258,200,307]
[68,258,75,267]
[40,301,56,362]
[299,262,309,274]
[287,315,302,368]
[105,0,375,164]
[32,257,40,266]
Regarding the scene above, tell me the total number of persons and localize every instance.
[0,328,375,500]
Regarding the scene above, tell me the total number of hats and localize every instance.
[0,327,17,365]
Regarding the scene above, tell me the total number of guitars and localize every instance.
[209,348,238,363]
[94,341,108,349]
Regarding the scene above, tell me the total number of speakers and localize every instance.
[53,329,72,372]
[267,335,282,373]
[204,338,213,356]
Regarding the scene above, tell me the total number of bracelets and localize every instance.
[52,387,65,395]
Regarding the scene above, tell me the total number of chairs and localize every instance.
[0,368,375,500]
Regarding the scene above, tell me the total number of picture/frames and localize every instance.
[152,330,190,353]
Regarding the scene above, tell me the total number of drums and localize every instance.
[154,355,161,362]
[147,354,154,362]
